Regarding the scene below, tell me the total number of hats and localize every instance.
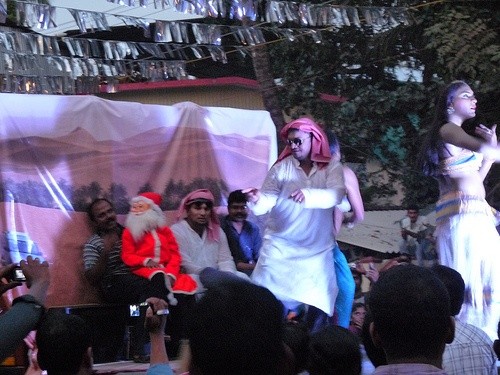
[131,192,163,218]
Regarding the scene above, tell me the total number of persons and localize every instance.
[415,80,500,344]
[0,113,500,375]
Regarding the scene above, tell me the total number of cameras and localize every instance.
[129,302,153,317]
[10,266,27,281]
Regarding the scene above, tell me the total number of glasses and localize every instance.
[286,136,309,147]
